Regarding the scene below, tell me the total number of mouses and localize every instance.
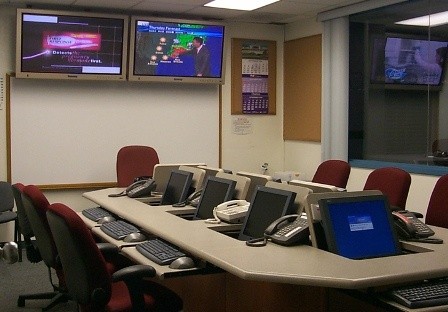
[97,216,117,224]
[169,256,195,269]
[122,232,147,242]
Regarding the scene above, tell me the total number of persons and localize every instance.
[170,37,209,78]
[414,46,448,86]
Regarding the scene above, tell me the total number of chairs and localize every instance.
[12,182,44,263]
[364,167,411,210]
[425,174,448,230]
[311,160,351,188]
[46,203,183,311]
[116,146,160,188]
[17,185,113,311]
[0,181,23,262]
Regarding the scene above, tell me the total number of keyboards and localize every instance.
[100,219,141,240]
[135,238,185,266]
[81,206,111,222]
[390,283,448,309]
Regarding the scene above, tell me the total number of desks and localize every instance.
[77,185,448,312]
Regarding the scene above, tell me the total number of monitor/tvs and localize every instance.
[237,184,297,240]
[128,15,229,84]
[15,8,129,81]
[192,175,237,220]
[160,170,193,205]
[318,194,403,260]
[367,32,448,91]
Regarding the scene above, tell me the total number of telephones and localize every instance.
[246,212,310,247]
[108,180,157,198]
[172,188,202,207]
[204,200,250,224]
[392,210,443,244]
